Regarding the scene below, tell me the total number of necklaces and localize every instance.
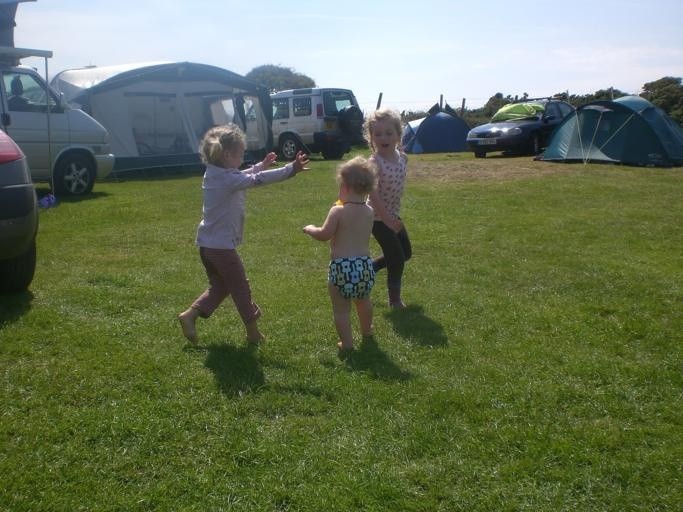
[342,201,366,206]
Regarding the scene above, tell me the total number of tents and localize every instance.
[398,110,474,156]
[534,94,681,169]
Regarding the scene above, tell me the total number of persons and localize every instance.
[353,109,412,312]
[302,156,375,354]
[177,123,312,347]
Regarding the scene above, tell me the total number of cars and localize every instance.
[0,111,38,294]
[464,94,576,159]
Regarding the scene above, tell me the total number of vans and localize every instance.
[0,61,115,198]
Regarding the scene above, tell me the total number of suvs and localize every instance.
[236,86,365,161]
